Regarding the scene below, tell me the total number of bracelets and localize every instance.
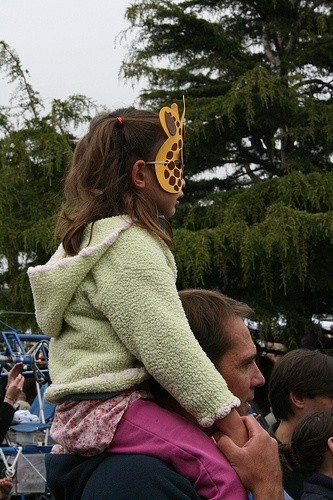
[6,396,17,402]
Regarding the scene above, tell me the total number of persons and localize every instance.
[26,94,249,500]
[0,362,25,447]
[265,348,333,500]
[44,289,283,500]
